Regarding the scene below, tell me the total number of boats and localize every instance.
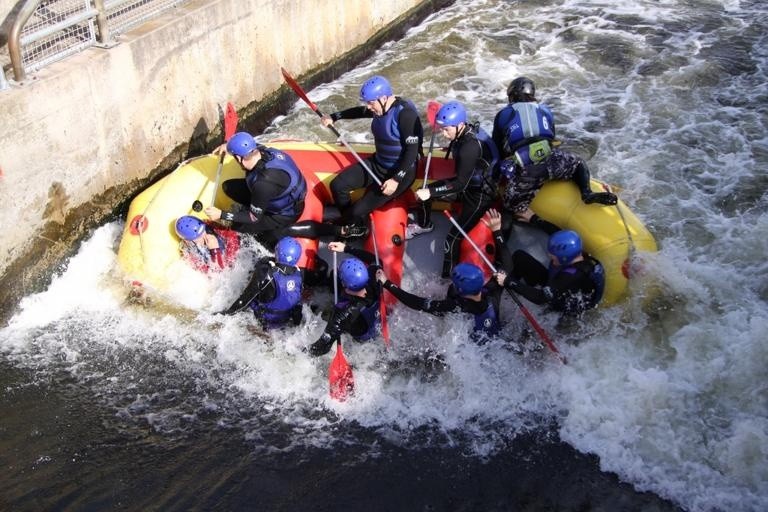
[120,141,660,334]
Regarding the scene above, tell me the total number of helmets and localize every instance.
[508,77,535,101]
[339,258,369,291]
[548,230,581,264]
[176,217,207,241]
[276,237,301,267]
[227,132,256,157]
[435,102,465,126]
[451,264,483,294]
[360,75,393,101]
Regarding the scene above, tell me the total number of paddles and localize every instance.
[422,101,444,189]
[329,251,355,402]
[281,67,382,186]
[210,103,238,207]
[370,214,390,347]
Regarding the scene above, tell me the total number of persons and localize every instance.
[490,77,617,211]
[405,102,500,287]
[174,215,370,275]
[375,209,514,347]
[321,77,423,228]
[206,131,307,249]
[306,242,385,358]
[493,208,605,314]
[210,238,329,330]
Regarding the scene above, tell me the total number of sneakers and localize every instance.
[341,224,370,239]
[405,223,433,239]
[584,192,617,205]
